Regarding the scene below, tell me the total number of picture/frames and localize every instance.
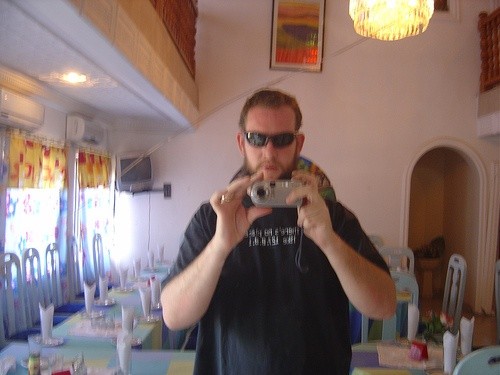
[269,0,326,72]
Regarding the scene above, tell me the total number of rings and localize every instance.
[222,195,233,203]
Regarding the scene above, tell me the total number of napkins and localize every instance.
[406,303,474,374]
[39,244,166,371]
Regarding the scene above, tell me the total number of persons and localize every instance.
[159,89,396,375]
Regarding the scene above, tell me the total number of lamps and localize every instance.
[347,0,434,41]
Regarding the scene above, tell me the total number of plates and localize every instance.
[20,259,171,368]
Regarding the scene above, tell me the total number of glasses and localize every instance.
[243,130,299,148]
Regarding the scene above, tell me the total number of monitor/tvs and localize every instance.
[115,154,154,193]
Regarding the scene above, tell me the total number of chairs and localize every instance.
[441,253,467,333]
[0,233,106,349]
[361,246,419,342]
[453,347,500,375]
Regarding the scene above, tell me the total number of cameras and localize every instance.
[251,179,304,208]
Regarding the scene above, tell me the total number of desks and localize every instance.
[0,260,196,375]
[349,338,465,375]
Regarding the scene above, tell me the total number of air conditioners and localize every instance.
[67,113,104,146]
[0,86,45,132]
